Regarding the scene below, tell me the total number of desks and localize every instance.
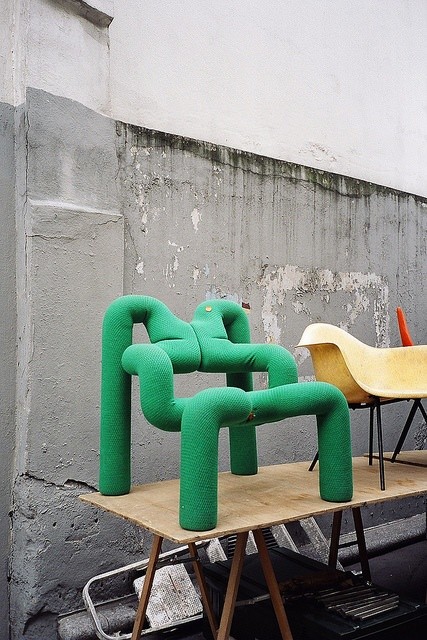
[78,449,427,640]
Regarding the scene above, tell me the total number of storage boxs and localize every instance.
[202,547,427,640]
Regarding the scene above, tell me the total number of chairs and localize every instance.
[97,293,427,530]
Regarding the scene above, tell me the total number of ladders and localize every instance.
[83,516,345,640]
